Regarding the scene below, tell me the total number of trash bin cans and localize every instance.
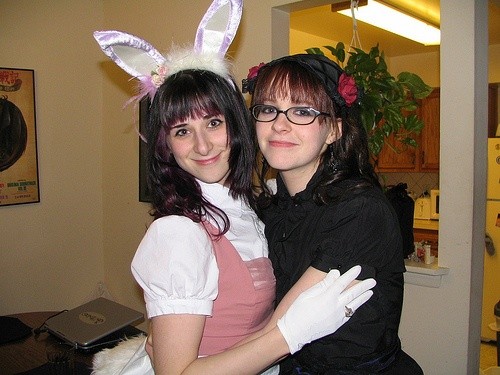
[487,300,500,366]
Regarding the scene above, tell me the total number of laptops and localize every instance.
[46,297,147,353]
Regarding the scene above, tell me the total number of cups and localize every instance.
[46,343,74,375]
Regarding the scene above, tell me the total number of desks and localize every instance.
[1,314,148,375]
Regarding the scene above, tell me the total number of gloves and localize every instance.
[278,265,377,356]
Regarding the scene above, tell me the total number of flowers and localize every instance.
[247,62,265,81]
[339,73,357,108]
[152,64,168,87]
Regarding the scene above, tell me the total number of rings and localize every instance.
[344,306,353,317]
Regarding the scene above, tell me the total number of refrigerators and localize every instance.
[481,135,500,344]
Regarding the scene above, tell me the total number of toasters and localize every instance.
[414,198,431,219]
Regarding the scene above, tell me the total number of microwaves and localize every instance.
[431,191,440,219]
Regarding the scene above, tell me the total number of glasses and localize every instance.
[248,104,333,125]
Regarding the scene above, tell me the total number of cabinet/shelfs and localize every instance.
[368,86,438,173]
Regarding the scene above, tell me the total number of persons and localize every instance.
[247,51,423,375]
[93,67,378,375]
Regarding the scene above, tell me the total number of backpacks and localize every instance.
[386,181,415,259]
